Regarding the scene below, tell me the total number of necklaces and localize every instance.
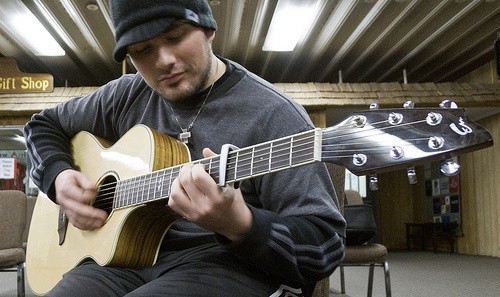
[162,55,218,144]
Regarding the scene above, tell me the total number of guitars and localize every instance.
[25,100,495,296]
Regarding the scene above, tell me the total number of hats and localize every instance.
[110,0,218,63]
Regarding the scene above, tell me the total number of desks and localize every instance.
[405,222,457,253]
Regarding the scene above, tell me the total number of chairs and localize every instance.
[338,204,391,297]
[0,190,27,297]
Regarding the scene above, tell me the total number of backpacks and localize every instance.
[345,204,376,245]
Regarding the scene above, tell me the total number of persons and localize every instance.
[23,0,347,297]
